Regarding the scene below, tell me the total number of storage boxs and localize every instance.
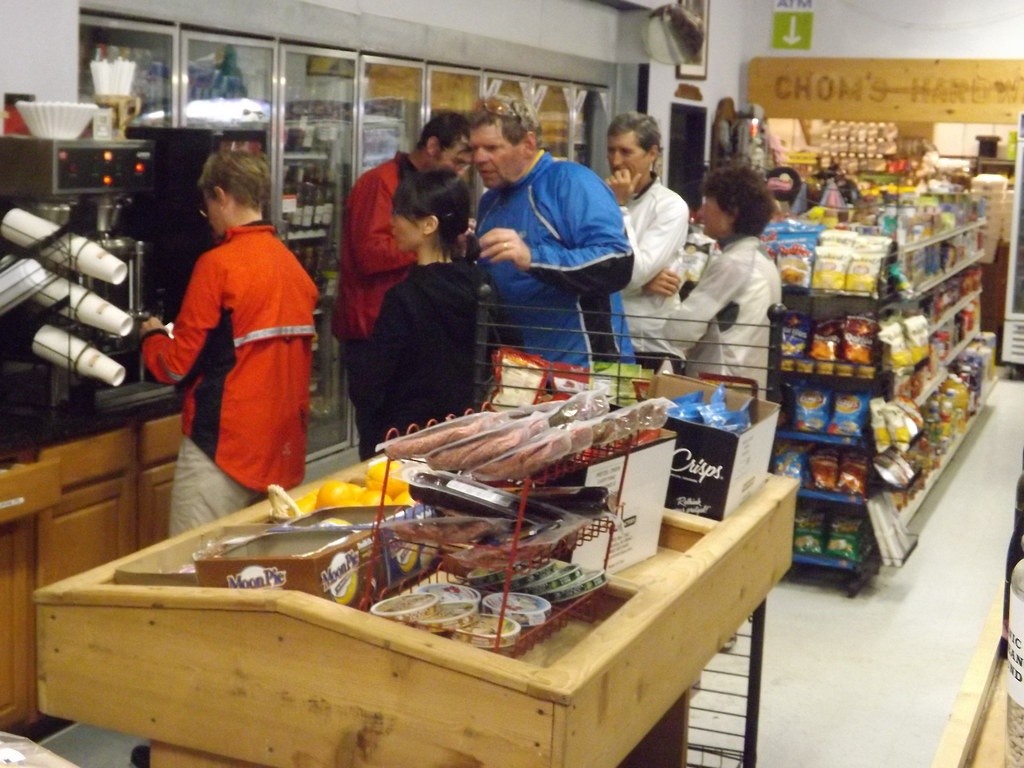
[267,505,415,588]
[529,429,676,575]
[609,371,782,527]
[196,529,389,610]
[971,174,1013,242]
[114,524,280,592]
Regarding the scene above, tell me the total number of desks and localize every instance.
[906,580,1024,768]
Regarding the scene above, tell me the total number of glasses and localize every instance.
[390,208,416,218]
[197,189,215,217]
[469,98,521,121]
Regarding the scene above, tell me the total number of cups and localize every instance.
[0,207,127,285]
[34,270,135,338]
[32,324,126,386]
[93,109,111,140]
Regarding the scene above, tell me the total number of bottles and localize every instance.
[283,165,334,229]
[291,248,341,282]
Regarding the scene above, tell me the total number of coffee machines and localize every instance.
[0,134,186,431]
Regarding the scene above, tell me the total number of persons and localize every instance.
[143,146,319,547]
[344,165,480,467]
[594,109,689,376]
[660,165,783,651]
[329,109,469,464]
[455,93,639,367]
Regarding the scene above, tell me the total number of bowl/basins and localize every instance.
[17,98,101,139]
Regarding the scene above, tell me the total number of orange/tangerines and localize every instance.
[287,459,418,517]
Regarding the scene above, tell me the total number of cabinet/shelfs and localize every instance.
[0,412,181,734]
[769,215,997,599]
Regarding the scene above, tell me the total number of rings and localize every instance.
[504,242,508,249]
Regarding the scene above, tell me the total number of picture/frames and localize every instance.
[675,0,711,80]
